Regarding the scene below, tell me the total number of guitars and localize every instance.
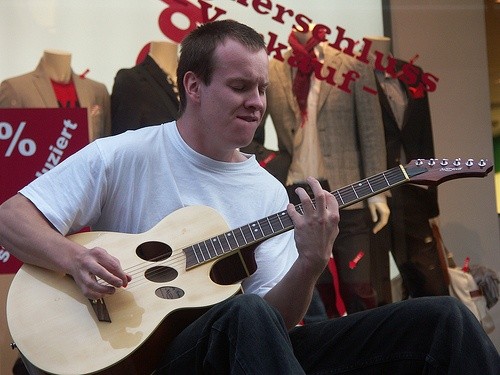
[5,157,495,375]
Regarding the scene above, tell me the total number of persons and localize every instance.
[1,49,110,144]
[253,23,394,319]
[362,34,451,308]
[0,20,500,375]
[109,41,183,135]
